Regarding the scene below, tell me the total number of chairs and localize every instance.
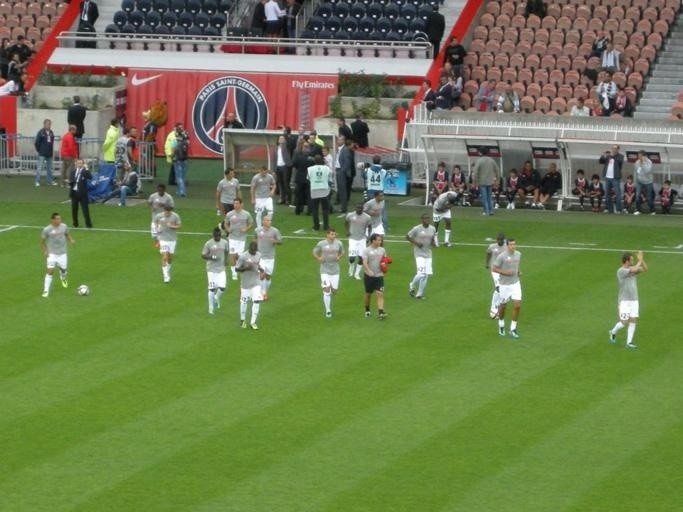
[105,0,235,37]
[449,0,683,121]
[300,0,444,42]
[0,0,71,51]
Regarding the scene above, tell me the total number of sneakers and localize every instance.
[407,283,425,298]
[39,279,68,297]
[433,240,451,248]
[162,272,171,282]
[488,310,520,336]
[262,294,267,299]
[234,273,240,280]
[496,200,657,218]
[607,330,639,350]
[35,180,40,186]
[349,271,358,278]
[204,300,388,328]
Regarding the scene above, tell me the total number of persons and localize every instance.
[344,202,372,281]
[632,149,658,216]
[471,145,501,217]
[601,43,624,74]
[333,132,352,214]
[336,141,358,205]
[34,118,58,188]
[420,79,433,103]
[215,168,244,235]
[249,166,276,227]
[172,127,191,198]
[504,167,519,210]
[335,116,352,141]
[351,110,370,148]
[233,241,266,331]
[154,204,182,283]
[587,173,605,212]
[571,169,589,210]
[68,159,93,229]
[606,249,650,350]
[608,185,617,203]
[622,174,636,214]
[492,238,522,339]
[128,126,140,170]
[201,227,229,316]
[490,175,503,210]
[101,118,120,164]
[118,162,142,207]
[66,95,87,155]
[465,163,480,207]
[484,231,508,318]
[475,78,497,113]
[276,124,334,230]
[449,164,467,198]
[435,62,454,91]
[164,122,190,185]
[362,189,392,272]
[360,155,393,235]
[658,180,679,214]
[424,4,445,58]
[611,87,631,118]
[432,190,458,248]
[433,73,452,110]
[0,34,38,96]
[537,162,562,209]
[224,198,253,281]
[518,160,541,209]
[570,97,590,117]
[584,30,610,61]
[147,183,175,248]
[250,0,268,40]
[449,67,463,108]
[253,214,282,300]
[361,232,388,320]
[442,36,468,82]
[598,143,624,214]
[59,124,79,188]
[633,188,657,212]
[224,112,245,130]
[313,227,345,318]
[429,161,449,195]
[283,0,303,54]
[39,212,76,299]
[405,213,437,301]
[597,70,617,116]
[264,0,288,42]
[495,84,520,114]
[76,0,100,30]
[112,128,136,180]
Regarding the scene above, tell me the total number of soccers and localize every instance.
[78,285,89,295]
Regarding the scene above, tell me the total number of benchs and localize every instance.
[427,171,683,216]
